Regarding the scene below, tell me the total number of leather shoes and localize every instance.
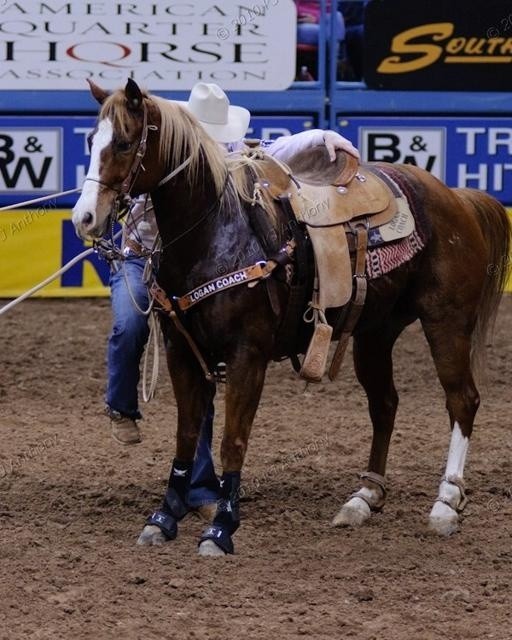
[195,502,221,521]
[110,410,142,445]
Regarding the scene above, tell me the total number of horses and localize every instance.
[70,77,512,558]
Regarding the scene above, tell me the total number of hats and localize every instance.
[182,82,252,141]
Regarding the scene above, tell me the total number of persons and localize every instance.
[295,0,367,64]
[103,83,361,524]
